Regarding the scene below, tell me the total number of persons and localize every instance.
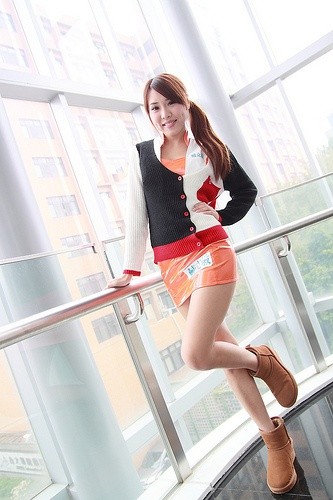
[102,74,300,493]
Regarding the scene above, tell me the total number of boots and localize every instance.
[258,415,298,495]
[247,345,298,408]
